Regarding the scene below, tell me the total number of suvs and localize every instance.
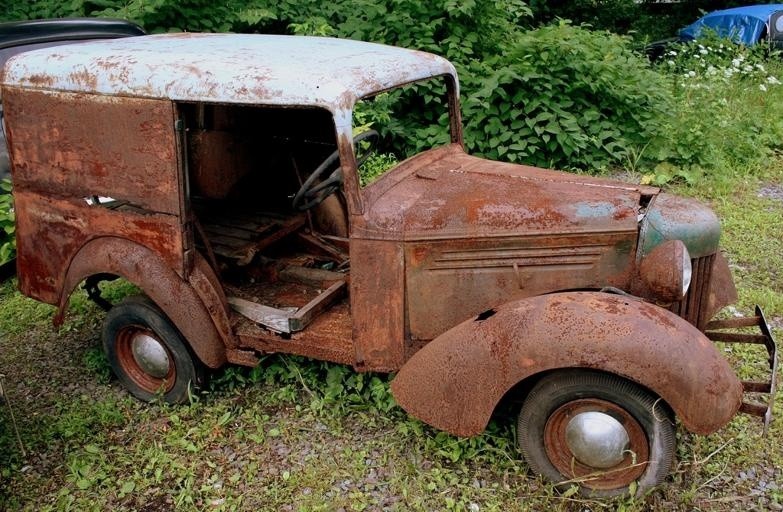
[0,27,778,503]
